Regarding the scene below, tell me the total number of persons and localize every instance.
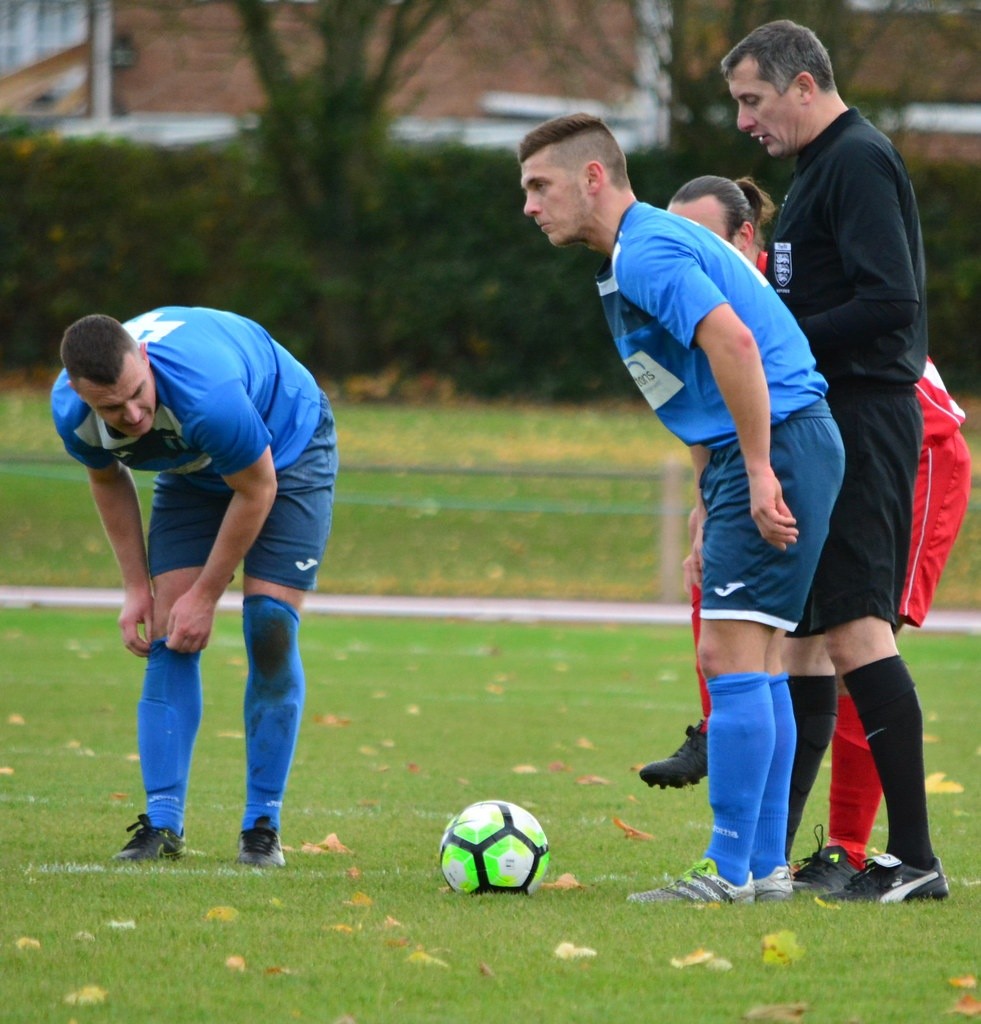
[517,114,844,906]
[640,175,971,891]
[719,18,952,902]
[48,304,340,870]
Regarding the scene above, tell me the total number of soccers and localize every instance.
[435,797,552,894]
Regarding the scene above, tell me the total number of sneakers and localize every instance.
[818,851,950,907]
[639,720,708,794]
[111,814,187,871]
[791,850,870,899]
[620,858,755,911]
[754,860,794,905]
[231,824,286,872]
